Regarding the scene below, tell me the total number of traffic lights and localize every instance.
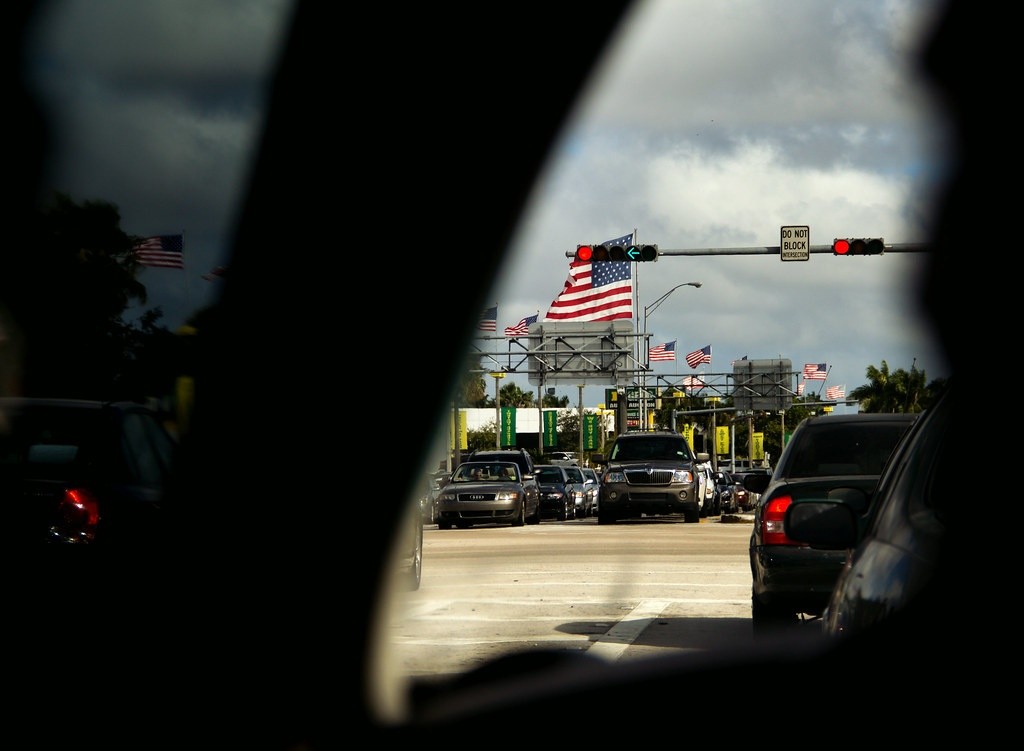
[576,245,659,262]
[832,238,885,256]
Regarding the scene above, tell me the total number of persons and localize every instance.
[472,470,485,480]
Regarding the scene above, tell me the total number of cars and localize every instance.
[383,430,772,592]
[752,358,1022,691]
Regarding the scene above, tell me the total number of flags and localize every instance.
[201,266,224,282]
[543,233,633,322]
[649,341,675,361]
[683,371,704,391]
[798,382,805,395]
[504,314,538,336]
[130,234,184,269]
[686,345,711,369]
[803,364,826,380]
[478,306,497,331]
[825,384,846,399]
[731,355,747,366]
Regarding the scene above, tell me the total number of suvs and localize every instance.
[467,447,544,526]
[591,432,711,525]
[2,398,181,565]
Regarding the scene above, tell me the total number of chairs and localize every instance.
[506,468,517,481]
[480,469,490,479]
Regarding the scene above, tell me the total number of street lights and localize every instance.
[644,282,702,431]
